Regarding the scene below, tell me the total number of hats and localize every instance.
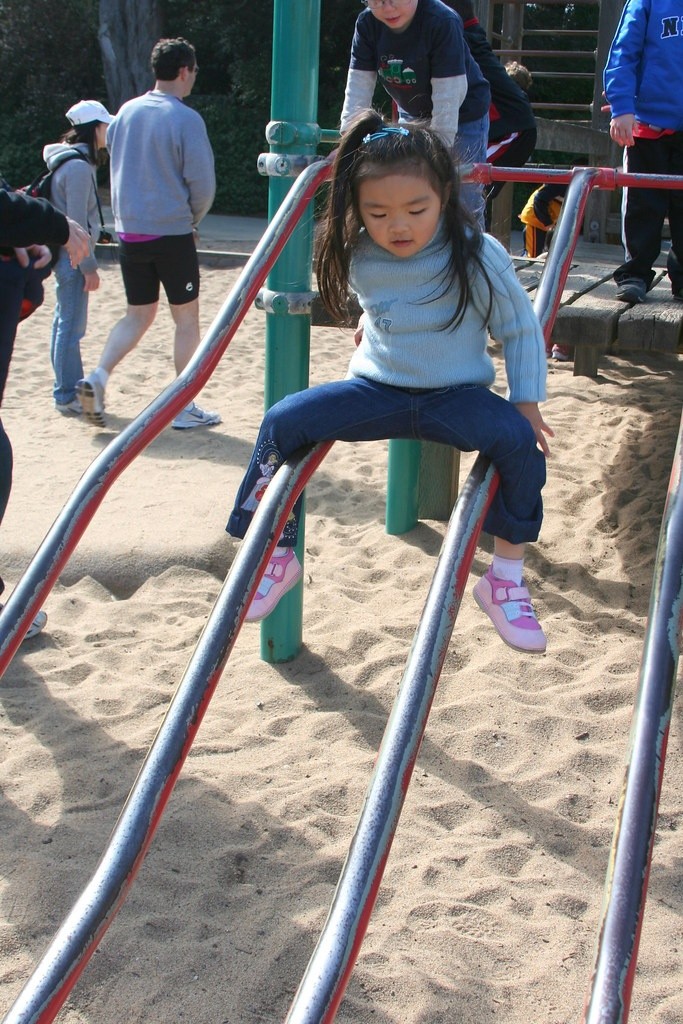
[64,99,118,126]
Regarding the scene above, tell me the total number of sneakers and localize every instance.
[244,548,300,622]
[75,371,108,427]
[671,289,683,299]
[615,275,646,302]
[171,404,221,428]
[55,393,84,413]
[25,611,48,639]
[473,564,546,652]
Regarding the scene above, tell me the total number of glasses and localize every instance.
[360,0,410,8]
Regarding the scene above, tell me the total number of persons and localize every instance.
[225,110,554,653]
[76,36,221,431]
[338,0,491,234]
[443,0,588,261]
[43,99,117,413]
[0,173,92,640]
[602,0,683,303]
